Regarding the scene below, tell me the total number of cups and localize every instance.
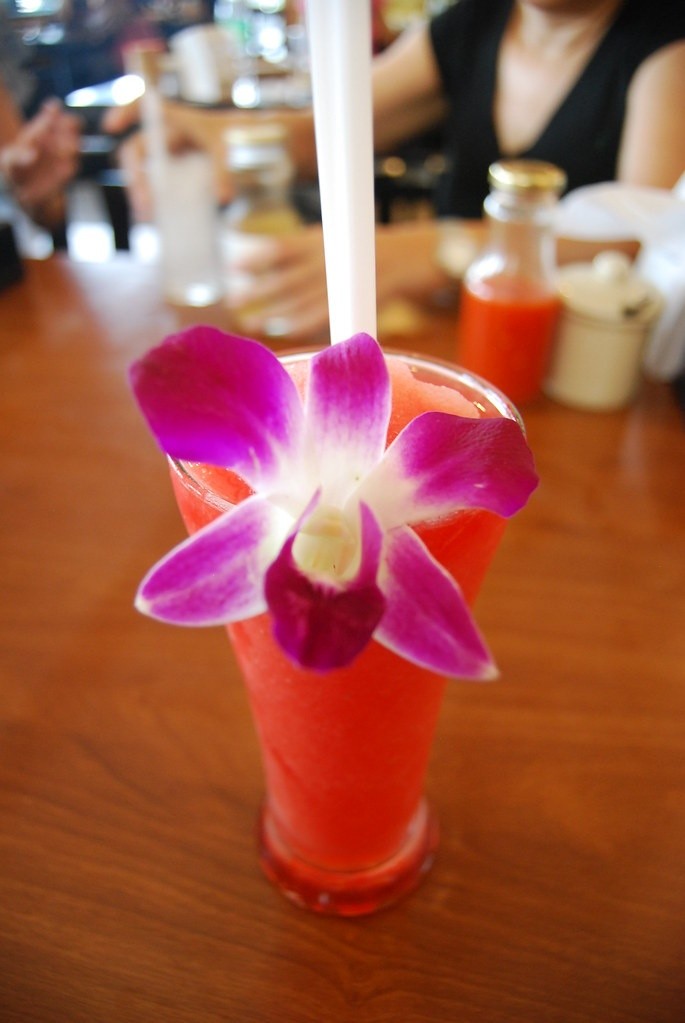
[133,27,260,307]
[543,246,660,415]
[162,343,527,920]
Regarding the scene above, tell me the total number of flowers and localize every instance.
[128,322,539,686]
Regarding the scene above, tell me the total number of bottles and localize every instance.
[213,124,308,342]
[455,159,569,409]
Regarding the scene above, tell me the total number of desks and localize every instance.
[0,213,685,1023]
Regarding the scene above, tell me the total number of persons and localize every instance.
[1,0,685,351]
